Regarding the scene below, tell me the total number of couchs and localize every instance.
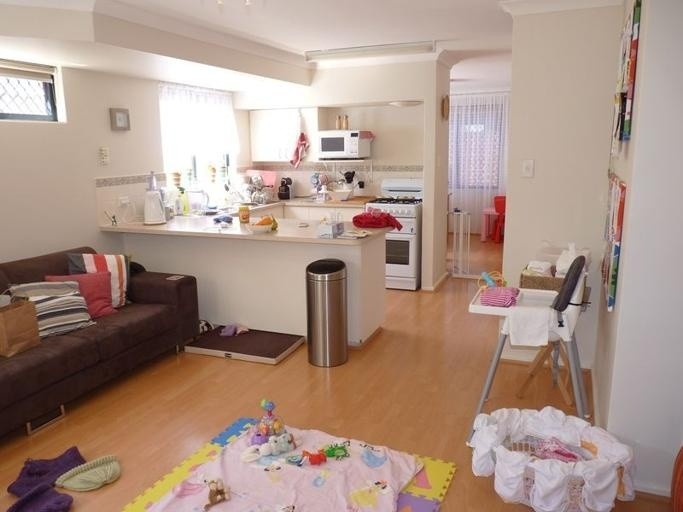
[1,245,200,440]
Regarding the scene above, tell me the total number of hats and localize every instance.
[55,455,121,492]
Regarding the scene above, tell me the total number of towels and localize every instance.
[507,305,552,346]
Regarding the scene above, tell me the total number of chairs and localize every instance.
[491,196,506,242]
[466,256,590,450]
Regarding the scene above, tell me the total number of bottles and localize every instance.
[336,116,342,130]
[175,195,184,216]
[239,207,249,223]
[342,115,348,130]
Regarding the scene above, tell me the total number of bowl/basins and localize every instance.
[329,189,352,200]
[245,223,273,233]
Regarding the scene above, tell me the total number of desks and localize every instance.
[481,209,505,242]
[517,283,592,405]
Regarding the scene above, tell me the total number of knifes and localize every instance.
[344,171,356,182]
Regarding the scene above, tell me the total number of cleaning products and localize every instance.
[176,195,183,215]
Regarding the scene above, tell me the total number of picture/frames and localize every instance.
[110,108,130,131]
[442,94,449,119]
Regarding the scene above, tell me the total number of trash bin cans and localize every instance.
[306,258,348,368]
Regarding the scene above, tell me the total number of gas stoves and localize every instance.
[367,197,422,215]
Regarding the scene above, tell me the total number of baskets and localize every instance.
[491,435,596,512]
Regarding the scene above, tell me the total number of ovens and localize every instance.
[382,217,418,278]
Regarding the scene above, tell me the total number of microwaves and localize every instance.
[316,130,371,159]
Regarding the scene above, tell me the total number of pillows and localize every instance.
[9,282,81,297]
[20,293,98,340]
[43,273,118,319]
[64,253,131,308]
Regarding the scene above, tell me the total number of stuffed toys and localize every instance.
[241,398,303,463]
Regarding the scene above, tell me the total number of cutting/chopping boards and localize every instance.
[327,195,376,204]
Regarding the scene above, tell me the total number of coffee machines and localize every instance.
[278,177,292,201]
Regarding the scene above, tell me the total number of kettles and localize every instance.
[143,191,166,225]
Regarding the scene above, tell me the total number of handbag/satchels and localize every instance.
[0,288,41,359]
